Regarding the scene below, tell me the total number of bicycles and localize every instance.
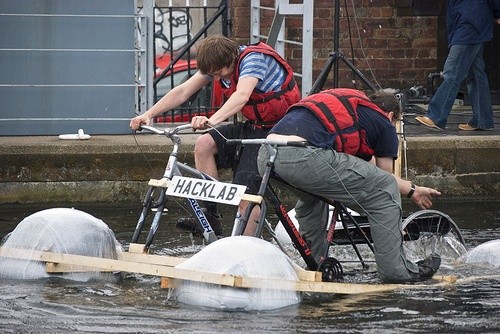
[223,136,468,282]
[127,114,249,255]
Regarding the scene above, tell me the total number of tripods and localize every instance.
[307,0,379,99]
